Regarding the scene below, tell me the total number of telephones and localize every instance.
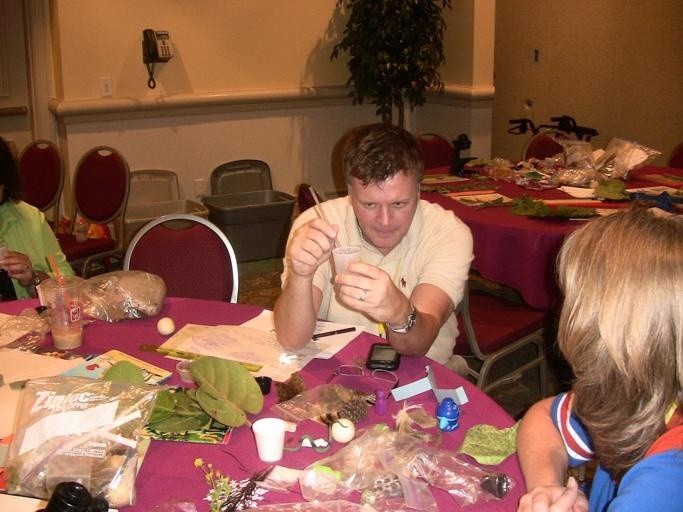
[142,28,174,63]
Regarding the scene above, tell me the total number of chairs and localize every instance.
[522,128,573,160]
[123,213,240,304]
[17,140,66,234]
[667,143,682,171]
[416,133,456,176]
[453,277,553,399]
[56,145,131,278]
[295,183,323,213]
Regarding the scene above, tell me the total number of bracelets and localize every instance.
[383,305,417,335]
[26,268,41,291]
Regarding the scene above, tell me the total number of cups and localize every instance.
[34,284,47,306]
[73,222,89,242]
[332,247,358,274]
[39,276,87,351]
[251,416,286,464]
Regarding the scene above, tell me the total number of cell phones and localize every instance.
[366,343,400,370]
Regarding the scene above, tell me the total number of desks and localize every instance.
[419,166,683,312]
[0,297,527,512]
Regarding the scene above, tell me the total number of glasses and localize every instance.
[326,364,398,406]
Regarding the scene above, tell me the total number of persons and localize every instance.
[514,205,682,511]
[271,122,475,375]
[0,137,78,298]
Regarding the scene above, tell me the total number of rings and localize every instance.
[361,289,367,302]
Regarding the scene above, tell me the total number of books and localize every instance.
[144,415,234,449]
[57,346,171,397]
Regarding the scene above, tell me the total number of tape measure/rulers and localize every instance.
[139,343,263,372]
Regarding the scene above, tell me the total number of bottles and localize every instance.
[374,390,385,415]
[543,157,554,170]
[435,398,461,433]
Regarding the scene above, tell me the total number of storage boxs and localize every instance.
[113,169,210,256]
[202,156,299,262]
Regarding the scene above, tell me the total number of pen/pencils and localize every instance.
[313,327,356,338]
